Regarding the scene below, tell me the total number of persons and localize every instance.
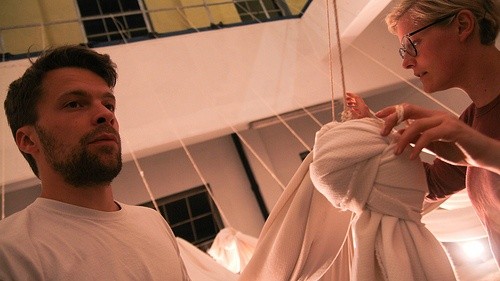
[0,44,190,281]
[346,0,500,269]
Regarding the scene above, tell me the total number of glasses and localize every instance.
[397,12,458,60]
